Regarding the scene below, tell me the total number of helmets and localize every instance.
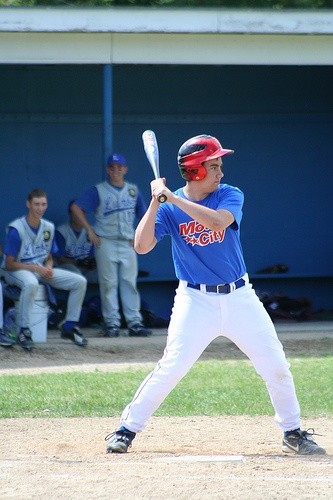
[177,135,234,182]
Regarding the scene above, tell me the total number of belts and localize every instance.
[187,279,245,295]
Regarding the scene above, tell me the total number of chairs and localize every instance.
[0,255,57,324]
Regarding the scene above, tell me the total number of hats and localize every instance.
[108,154,128,166]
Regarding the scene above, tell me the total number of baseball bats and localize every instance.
[141,129,167,204]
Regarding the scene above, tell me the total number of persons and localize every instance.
[0,189,88,350]
[104,134,326,456]
[47,201,99,327]
[71,153,152,337]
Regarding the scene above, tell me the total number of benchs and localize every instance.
[136,273,333,281]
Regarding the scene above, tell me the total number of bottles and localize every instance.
[268,303,278,311]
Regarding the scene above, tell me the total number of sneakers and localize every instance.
[0,331,16,345]
[61,323,89,346]
[105,427,135,453]
[281,427,326,455]
[104,325,119,337]
[128,326,151,336]
[16,330,36,349]
